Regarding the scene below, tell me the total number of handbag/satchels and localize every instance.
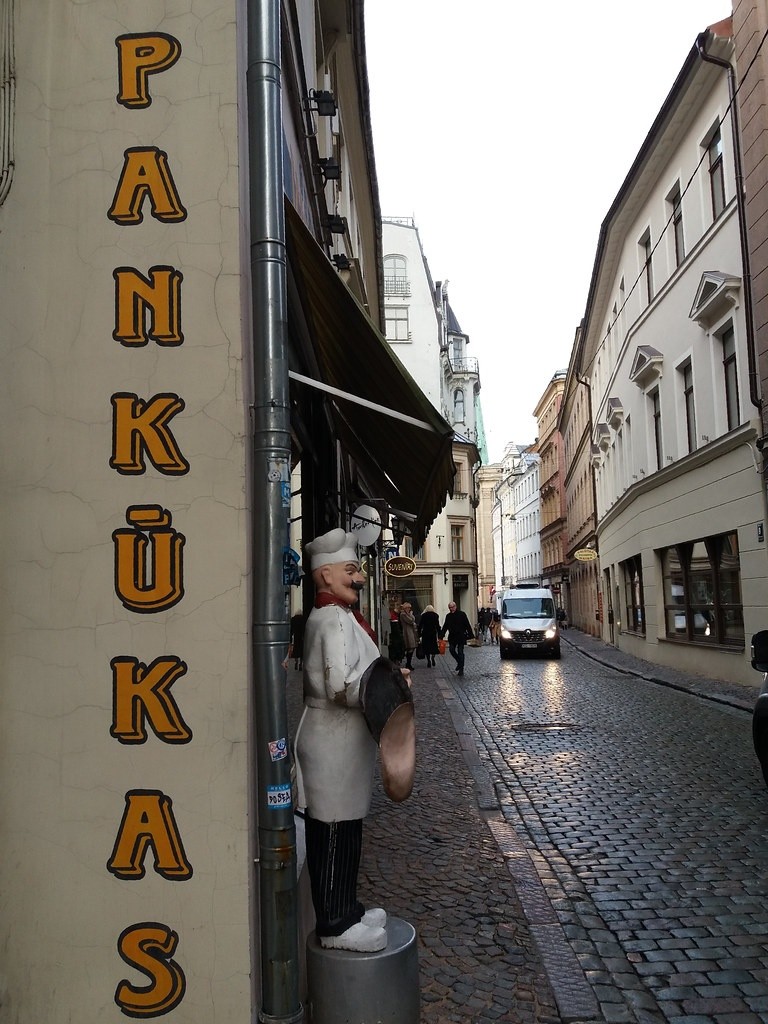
[416,643,426,660]
[437,638,447,655]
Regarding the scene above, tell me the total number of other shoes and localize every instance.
[405,663,414,671]
[427,661,431,668]
[432,659,436,667]
[455,664,460,671]
[457,669,465,676]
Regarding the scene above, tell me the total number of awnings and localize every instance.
[289,195,457,556]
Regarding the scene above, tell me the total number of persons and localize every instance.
[417,605,442,668]
[478,606,501,645]
[290,608,305,671]
[556,607,569,630]
[438,601,476,676]
[400,602,418,671]
[293,526,414,952]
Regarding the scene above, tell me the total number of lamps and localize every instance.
[702,435,708,441]
[632,475,637,478]
[508,498,538,520]
[640,468,645,474]
[303,89,336,115]
[666,456,673,460]
[329,213,348,235]
[318,157,341,179]
[331,254,351,272]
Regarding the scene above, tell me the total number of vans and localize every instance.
[670,584,711,635]
[499,581,561,660]
